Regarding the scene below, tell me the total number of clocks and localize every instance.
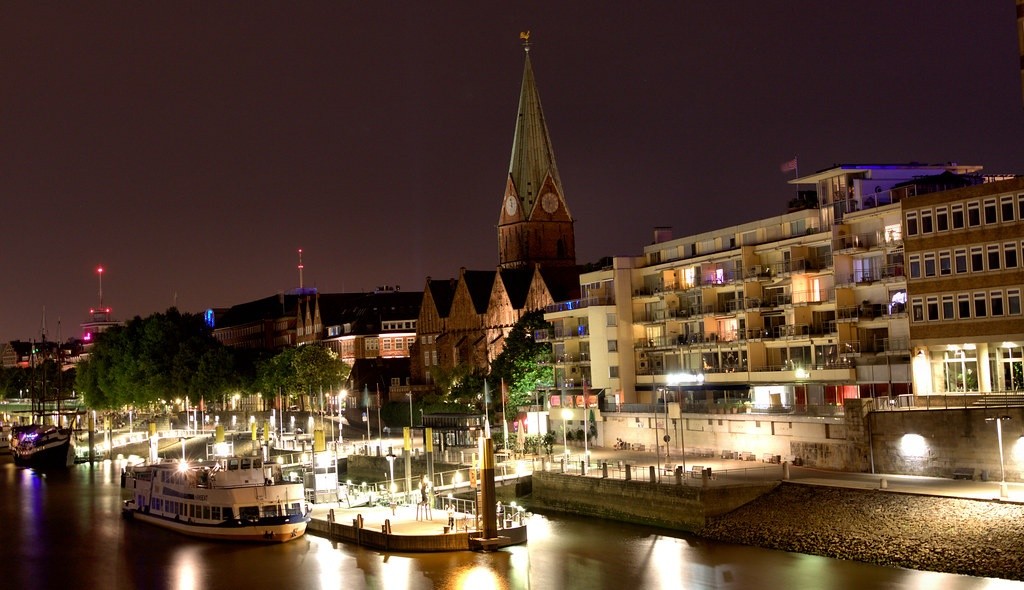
[542,193,559,214]
[505,196,517,217]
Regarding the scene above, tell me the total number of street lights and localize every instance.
[563,409,573,467]
[986,415,1010,498]
[386,454,401,517]
[335,388,349,447]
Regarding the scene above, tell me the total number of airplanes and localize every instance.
[119,450,313,544]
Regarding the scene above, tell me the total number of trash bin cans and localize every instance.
[678,465,682,474]
[707,467,712,477]
[618,460,622,469]
[597,458,601,467]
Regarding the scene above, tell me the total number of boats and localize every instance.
[6,425,73,472]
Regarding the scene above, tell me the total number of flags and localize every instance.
[779,159,798,172]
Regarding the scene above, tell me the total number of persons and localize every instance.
[447,503,457,528]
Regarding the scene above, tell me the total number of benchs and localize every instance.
[738,452,752,460]
[686,465,704,478]
[761,453,773,463]
[614,442,626,450]
[631,443,642,451]
[780,455,796,465]
[646,444,657,452]
[718,449,731,459]
[664,464,677,475]
